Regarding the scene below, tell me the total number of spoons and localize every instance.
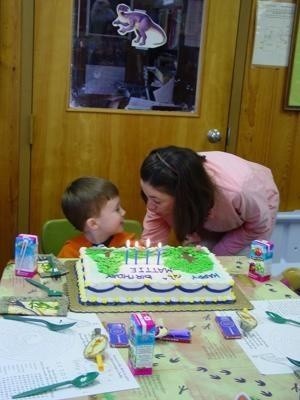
[3,313,79,332]
[45,251,61,283]
[12,368,98,399]
[23,275,67,300]
[266,307,300,328]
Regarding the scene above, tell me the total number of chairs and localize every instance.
[42,217,143,258]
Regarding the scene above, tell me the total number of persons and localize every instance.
[139,146,280,257]
[58,177,138,259]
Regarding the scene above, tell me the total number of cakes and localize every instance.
[73,246,237,306]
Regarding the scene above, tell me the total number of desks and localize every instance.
[1,251,299,399]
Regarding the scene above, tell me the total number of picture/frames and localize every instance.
[280,2,300,115]
[64,0,211,119]
